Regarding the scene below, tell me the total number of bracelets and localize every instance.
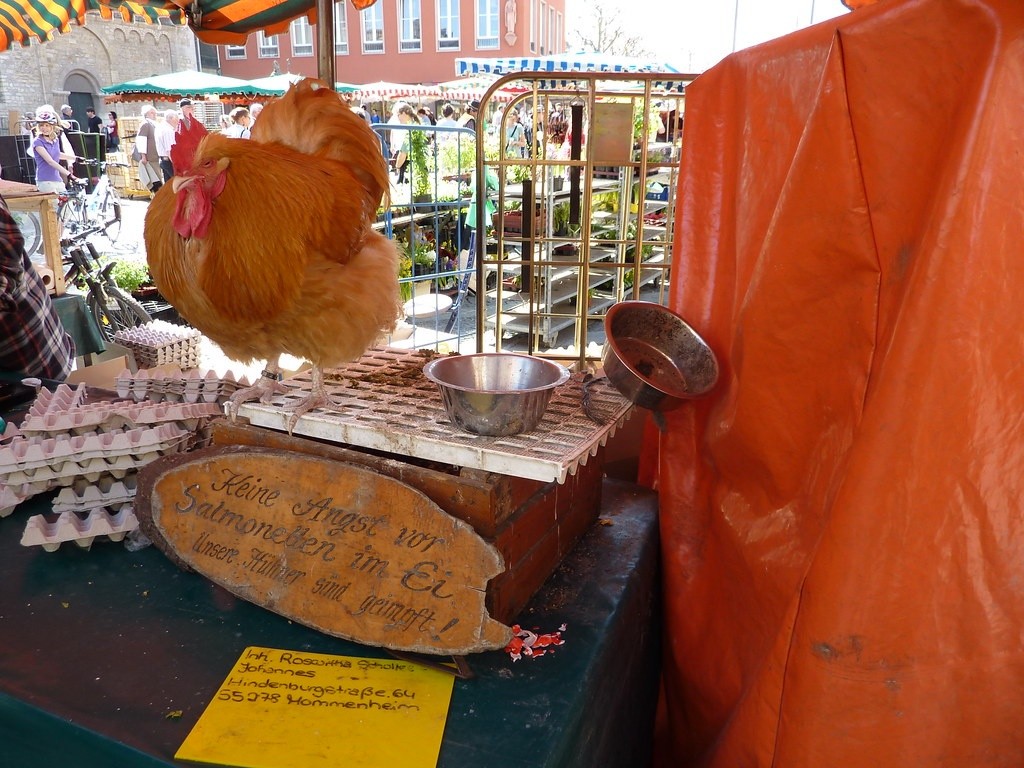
[66,173,77,182]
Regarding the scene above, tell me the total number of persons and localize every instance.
[645,100,683,144]
[350,99,569,186]
[33,113,86,256]
[22,104,119,236]
[133,98,193,197]
[220,102,265,140]
[0,193,78,419]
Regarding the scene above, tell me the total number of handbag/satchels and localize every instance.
[132,148,142,162]
[102,127,112,148]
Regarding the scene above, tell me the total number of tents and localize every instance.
[455,46,686,92]
[97,70,532,111]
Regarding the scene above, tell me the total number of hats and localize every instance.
[180,97,196,107]
[468,100,480,110]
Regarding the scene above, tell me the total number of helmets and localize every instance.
[36,112,59,127]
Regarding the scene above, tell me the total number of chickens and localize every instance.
[145,76,406,439]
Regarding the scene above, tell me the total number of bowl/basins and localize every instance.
[423,352,571,437]
[600,299,720,413]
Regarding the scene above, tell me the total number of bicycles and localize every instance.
[38,201,154,347]
[56,155,124,262]
